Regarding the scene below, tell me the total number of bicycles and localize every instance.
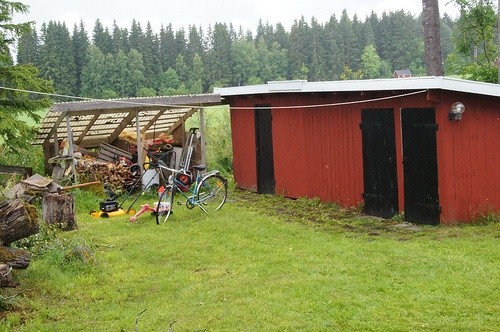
[150,160,229,225]
